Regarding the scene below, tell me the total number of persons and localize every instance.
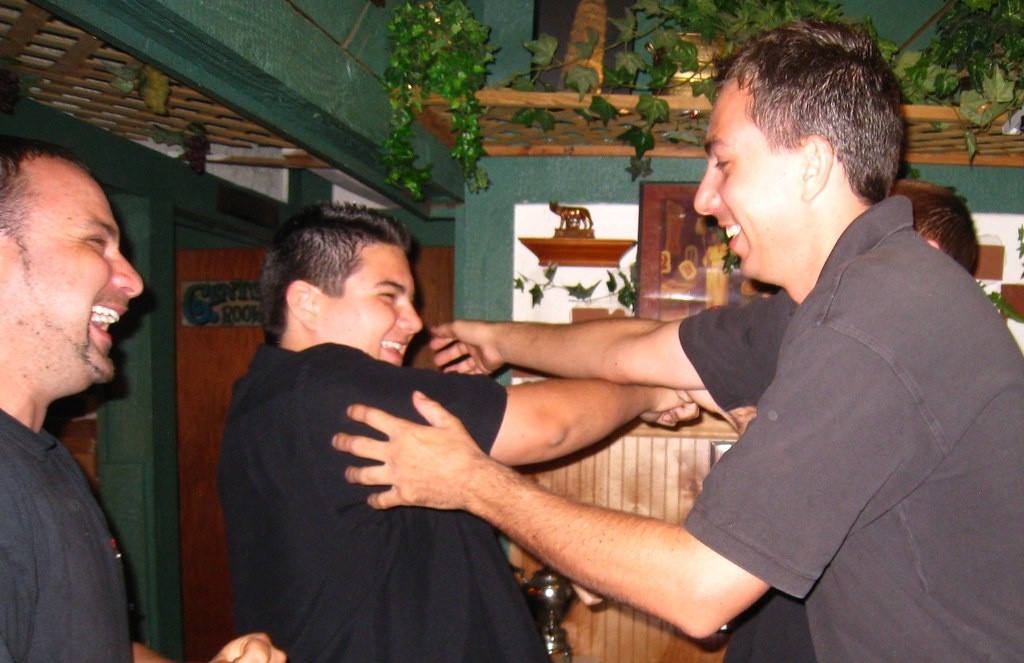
[217,203,699,663]
[0,136,287,663]
[331,19,1024,663]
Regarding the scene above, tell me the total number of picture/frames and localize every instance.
[635,180,780,321]
